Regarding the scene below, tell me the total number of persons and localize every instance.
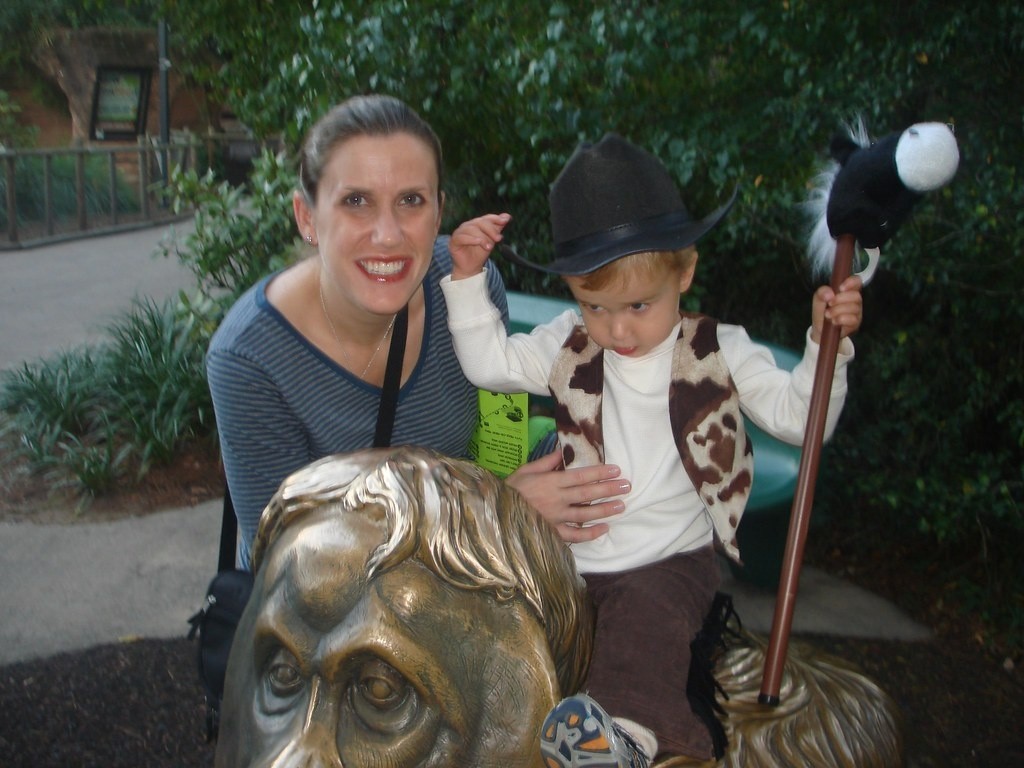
[205,96,633,569]
[441,129,862,768]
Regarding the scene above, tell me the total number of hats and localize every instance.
[499,132,740,277]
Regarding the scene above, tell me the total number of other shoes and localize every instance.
[187,569,256,707]
[540,694,652,768]
